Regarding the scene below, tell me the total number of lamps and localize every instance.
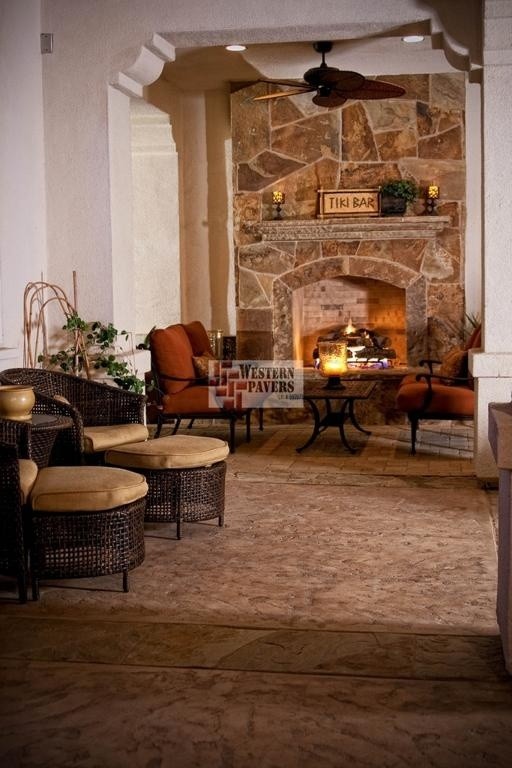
[316,342,349,389]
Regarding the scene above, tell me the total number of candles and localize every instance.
[428,186,439,199]
[272,192,283,204]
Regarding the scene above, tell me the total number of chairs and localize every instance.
[0,418,38,604]
[0,368,149,466]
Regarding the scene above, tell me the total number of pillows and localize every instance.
[439,323,482,386]
[150,321,223,394]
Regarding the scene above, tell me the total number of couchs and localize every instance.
[150,349,275,453]
[397,360,482,456]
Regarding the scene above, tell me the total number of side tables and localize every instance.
[21,413,74,471]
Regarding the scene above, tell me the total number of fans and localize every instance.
[253,42,406,107]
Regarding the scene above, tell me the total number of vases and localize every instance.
[0,384,35,421]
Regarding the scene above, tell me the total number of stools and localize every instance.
[30,464,149,599]
[105,435,229,540]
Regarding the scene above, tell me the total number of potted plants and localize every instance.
[381,179,420,216]
